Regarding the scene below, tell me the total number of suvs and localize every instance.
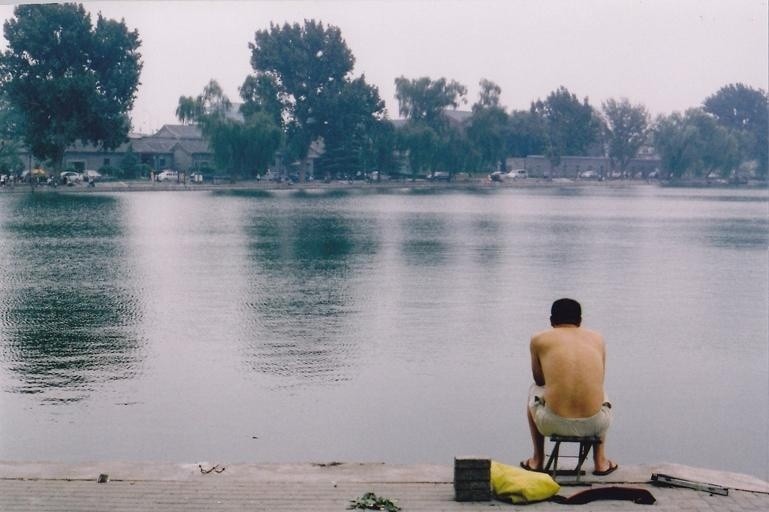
[154,169,184,183]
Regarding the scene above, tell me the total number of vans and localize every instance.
[507,168,529,180]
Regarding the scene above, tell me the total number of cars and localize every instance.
[367,171,395,183]
[489,171,509,181]
[0,167,105,186]
[579,171,606,181]
[427,171,458,184]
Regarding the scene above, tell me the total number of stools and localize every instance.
[543,436,602,487]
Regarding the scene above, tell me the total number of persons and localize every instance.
[520,298,617,475]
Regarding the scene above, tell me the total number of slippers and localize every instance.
[592,459,619,475]
[520,459,542,471]
